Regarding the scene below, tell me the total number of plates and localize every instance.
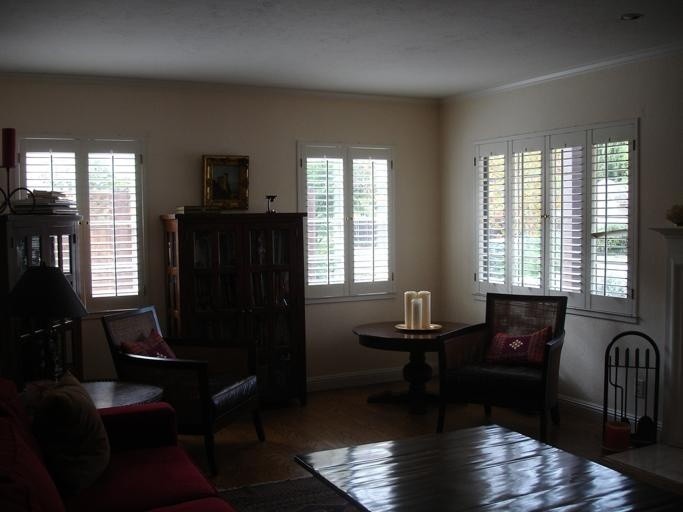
[393,323,444,332]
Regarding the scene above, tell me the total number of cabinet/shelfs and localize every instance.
[160,212,308,407]
[1,215,84,377]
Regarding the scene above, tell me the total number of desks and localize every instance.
[292,424,683,512]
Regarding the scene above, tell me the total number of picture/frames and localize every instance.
[202,155,249,210]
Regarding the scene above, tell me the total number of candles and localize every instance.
[1,128,16,167]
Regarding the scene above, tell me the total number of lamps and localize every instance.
[5,261,89,379]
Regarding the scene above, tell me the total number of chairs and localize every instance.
[101,304,265,474]
[436,292,568,441]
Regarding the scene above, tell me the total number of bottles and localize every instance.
[410,298,422,329]
[418,291,431,328]
[403,291,417,328]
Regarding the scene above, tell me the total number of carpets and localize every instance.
[219,456,683,512]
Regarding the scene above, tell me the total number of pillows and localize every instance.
[484,326,552,368]
[33,370,110,497]
[119,325,175,357]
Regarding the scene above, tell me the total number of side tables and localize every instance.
[352,320,470,415]
[79,381,163,409]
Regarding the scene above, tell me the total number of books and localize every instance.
[12,191,79,215]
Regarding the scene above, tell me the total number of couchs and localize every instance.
[0,378,236,511]
